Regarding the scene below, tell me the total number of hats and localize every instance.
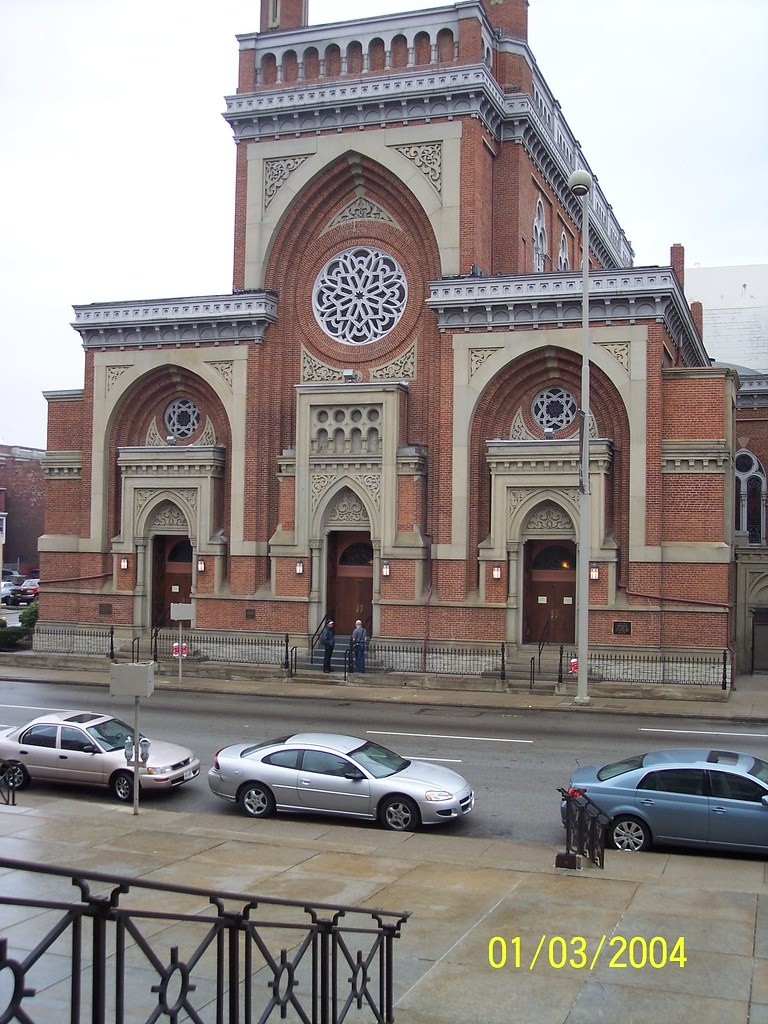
[325,620,333,624]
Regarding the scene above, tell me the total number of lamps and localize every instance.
[492,561,502,580]
[342,368,359,383]
[590,562,600,580]
[167,435,177,446]
[295,559,303,575]
[545,429,556,439]
[197,557,205,571]
[382,560,390,577]
[121,554,128,570]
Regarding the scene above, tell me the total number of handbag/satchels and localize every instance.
[354,642,359,649]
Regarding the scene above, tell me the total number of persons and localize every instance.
[319,619,335,674]
[352,619,367,674]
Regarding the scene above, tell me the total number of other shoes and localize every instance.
[354,669,365,673]
[324,668,334,672]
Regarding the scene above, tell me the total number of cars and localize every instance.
[559,748,768,854]
[0,711,201,803]
[208,732,474,832]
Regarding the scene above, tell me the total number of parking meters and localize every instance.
[125,737,151,816]
[172,643,187,683]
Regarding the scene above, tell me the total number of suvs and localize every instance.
[1,569,40,606]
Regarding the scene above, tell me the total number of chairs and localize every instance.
[713,771,734,799]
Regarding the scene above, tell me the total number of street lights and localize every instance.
[566,169,594,705]
